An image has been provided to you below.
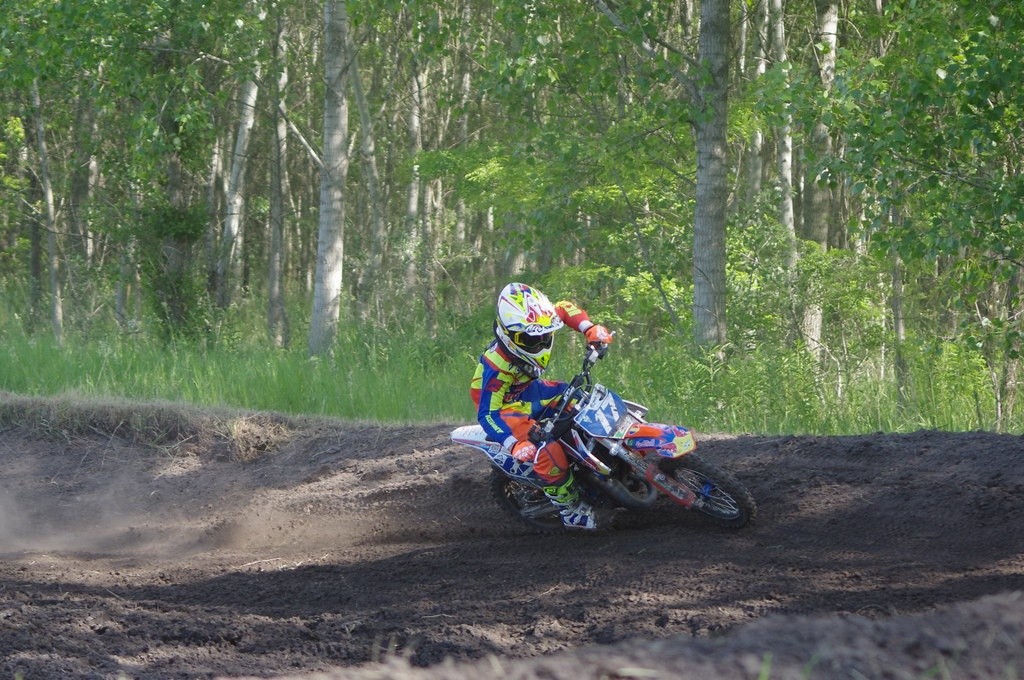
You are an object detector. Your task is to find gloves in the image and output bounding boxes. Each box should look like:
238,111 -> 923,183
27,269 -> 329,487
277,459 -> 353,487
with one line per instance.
511,440 -> 537,462
584,324 -> 613,344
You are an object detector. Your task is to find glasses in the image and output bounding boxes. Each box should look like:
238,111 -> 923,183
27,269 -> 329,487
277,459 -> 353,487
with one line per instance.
515,330 -> 555,348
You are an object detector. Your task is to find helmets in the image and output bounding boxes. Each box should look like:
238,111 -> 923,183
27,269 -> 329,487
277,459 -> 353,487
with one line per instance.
493,283 -> 564,370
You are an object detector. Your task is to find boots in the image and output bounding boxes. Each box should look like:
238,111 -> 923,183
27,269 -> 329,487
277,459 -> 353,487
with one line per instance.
543,470 -> 617,531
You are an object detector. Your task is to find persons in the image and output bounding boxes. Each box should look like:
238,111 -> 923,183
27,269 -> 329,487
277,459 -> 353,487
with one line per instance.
469,282 -> 612,532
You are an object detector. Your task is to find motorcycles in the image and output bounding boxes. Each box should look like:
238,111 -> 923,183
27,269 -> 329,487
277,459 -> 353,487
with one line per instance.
450,330 -> 758,537
